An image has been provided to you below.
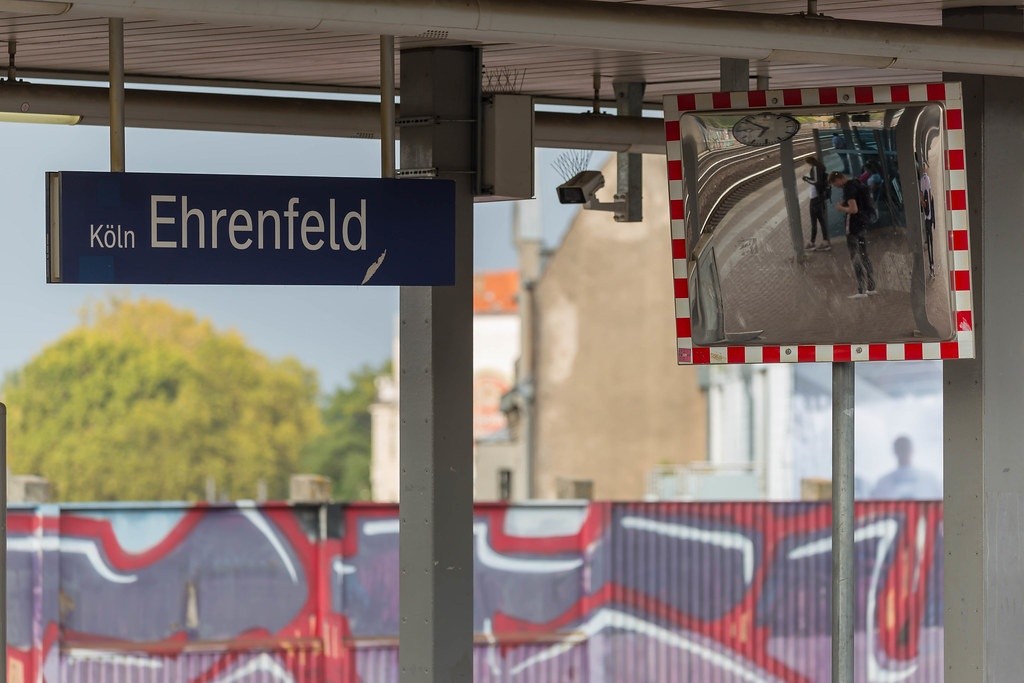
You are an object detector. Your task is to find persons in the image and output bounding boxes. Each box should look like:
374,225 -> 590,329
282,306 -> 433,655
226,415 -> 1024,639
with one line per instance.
921,164 -> 935,279
871,436 -> 943,500
833,131 -> 851,174
829,170 -> 879,297
802,156 -> 832,251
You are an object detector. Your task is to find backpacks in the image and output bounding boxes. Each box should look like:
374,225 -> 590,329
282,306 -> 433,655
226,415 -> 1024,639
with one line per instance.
850,181 -> 879,225
815,170 -> 831,201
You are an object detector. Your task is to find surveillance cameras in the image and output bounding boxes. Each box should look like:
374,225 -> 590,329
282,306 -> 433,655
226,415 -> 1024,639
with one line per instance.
557,171 -> 604,204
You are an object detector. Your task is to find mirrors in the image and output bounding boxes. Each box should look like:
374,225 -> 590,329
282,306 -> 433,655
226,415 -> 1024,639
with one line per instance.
663,81 -> 976,365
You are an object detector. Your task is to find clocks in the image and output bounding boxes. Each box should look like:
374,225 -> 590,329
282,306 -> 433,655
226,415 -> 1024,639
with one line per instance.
732,113 -> 799,147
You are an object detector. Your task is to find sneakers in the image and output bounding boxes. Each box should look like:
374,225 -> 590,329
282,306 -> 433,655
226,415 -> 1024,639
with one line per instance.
866,290 -> 877,296
803,243 -> 816,251
849,293 -> 867,300
815,243 -> 831,251
